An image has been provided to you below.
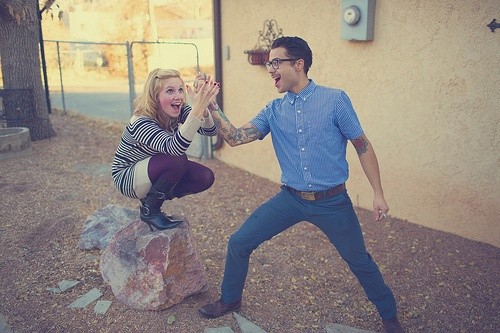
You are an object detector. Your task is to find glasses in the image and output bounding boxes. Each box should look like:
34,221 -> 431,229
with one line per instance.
264,58 -> 299,71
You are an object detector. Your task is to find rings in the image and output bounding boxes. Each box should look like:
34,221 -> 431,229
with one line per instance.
381,213 -> 387,219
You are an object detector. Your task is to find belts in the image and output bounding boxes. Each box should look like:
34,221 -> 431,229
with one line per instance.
287,182 -> 346,201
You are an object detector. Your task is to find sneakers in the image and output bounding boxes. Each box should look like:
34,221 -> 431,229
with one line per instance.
383,316 -> 404,333
197,298 -> 241,319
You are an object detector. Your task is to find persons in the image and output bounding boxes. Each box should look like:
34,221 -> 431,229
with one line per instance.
112,68 -> 221,232
193,37 -> 405,333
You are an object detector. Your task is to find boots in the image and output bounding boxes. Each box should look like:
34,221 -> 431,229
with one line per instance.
140,184 -> 182,232
